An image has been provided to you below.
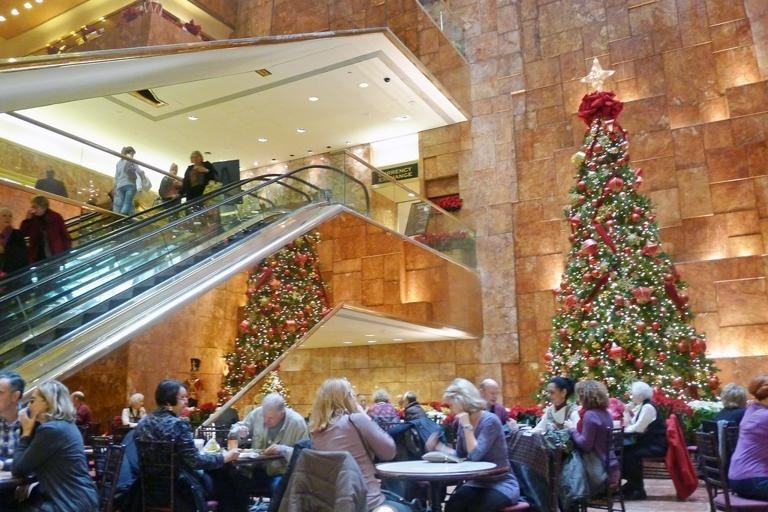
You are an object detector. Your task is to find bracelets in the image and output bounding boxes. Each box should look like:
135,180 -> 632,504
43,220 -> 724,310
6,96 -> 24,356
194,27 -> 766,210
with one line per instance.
462,424 -> 473,430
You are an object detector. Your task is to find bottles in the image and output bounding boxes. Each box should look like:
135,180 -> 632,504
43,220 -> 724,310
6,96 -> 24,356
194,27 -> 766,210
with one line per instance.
227,430 -> 239,451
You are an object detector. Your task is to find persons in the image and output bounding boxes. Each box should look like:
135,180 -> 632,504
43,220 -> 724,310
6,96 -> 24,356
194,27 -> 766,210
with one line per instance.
531,377 -> 666,512
479,378 -> 517,430
425,377 -> 520,512
308,378 -> 419,512
1,372 -> 100,512
0,207 -> 31,317
182,150 -> 218,230
36,167 -> 69,199
112,145 -> 147,215
706,375 -> 766,501
158,162 -> 183,223
20,195 -> 71,310
357,389 -> 428,423
110,379 -> 309,512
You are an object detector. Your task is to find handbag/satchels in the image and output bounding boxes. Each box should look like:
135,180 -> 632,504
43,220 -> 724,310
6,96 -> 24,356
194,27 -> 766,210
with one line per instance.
421,451 -> 466,463
201,180 -> 224,206
124,162 -> 137,181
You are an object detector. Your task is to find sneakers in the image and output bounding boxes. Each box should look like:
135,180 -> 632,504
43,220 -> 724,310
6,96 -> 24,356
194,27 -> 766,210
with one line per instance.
613,483 -> 645,499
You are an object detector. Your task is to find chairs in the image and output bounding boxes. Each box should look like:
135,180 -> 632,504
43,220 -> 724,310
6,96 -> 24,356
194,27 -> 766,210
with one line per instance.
135,438 -> 176,512
501,419 -> 768,512
89,436 -> 127,512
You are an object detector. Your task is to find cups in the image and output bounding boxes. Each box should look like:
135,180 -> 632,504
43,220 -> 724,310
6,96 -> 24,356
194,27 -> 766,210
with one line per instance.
613,420 -> 621,427
193,438 -> 204,451
